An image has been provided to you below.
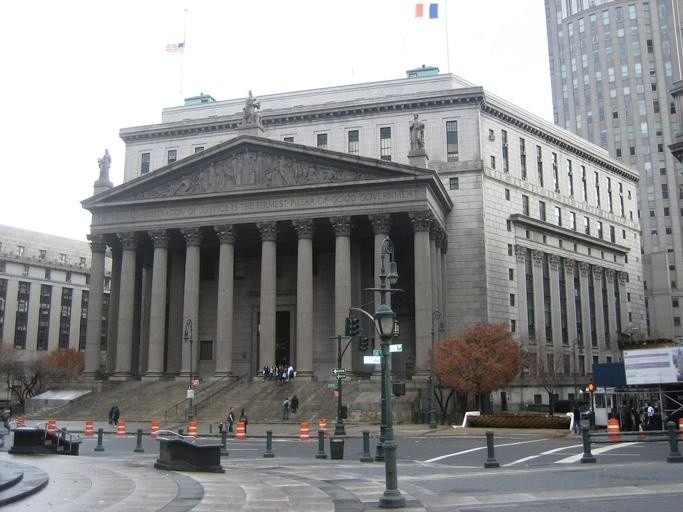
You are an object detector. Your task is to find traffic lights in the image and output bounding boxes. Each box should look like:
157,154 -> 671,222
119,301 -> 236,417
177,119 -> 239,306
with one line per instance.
587,383 -> 594,392
349,316 -> 359,336
362,337 -> 368,351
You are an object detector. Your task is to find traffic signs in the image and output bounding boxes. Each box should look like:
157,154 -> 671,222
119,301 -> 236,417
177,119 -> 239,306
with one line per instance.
330,368 -> 344,376
327,384 -> 339,389
335,373 -> 345,380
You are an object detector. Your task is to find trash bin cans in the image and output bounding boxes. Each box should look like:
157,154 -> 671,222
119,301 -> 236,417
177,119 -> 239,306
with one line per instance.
330,436 -> 344,459
417,412 -> 424,425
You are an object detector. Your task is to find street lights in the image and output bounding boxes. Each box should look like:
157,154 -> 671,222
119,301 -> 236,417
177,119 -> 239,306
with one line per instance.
572,338 -> 584,410
362,237 -> 405,463
183,318 -> 193,422
372,304 -> 406,510
429,309 -> 445,427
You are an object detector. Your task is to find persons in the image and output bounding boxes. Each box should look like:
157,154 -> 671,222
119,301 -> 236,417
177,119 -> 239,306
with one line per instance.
228,407 -> 235,432
621,400 -> 656,431
99,149 -> 112,179
282,397 -> 290,420
262,362 -> 296,382
410,113 -> 425,150
114,406 -> 120,425
239,408 -> 248,432
108,406 -> 115,424
245,90 -> 256,123
291,396 -> 299,413
571,404 -> 581,436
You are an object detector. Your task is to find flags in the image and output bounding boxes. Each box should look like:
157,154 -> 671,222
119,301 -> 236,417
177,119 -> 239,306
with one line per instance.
168,41 -> 185,55
416,3 -> 446,19
